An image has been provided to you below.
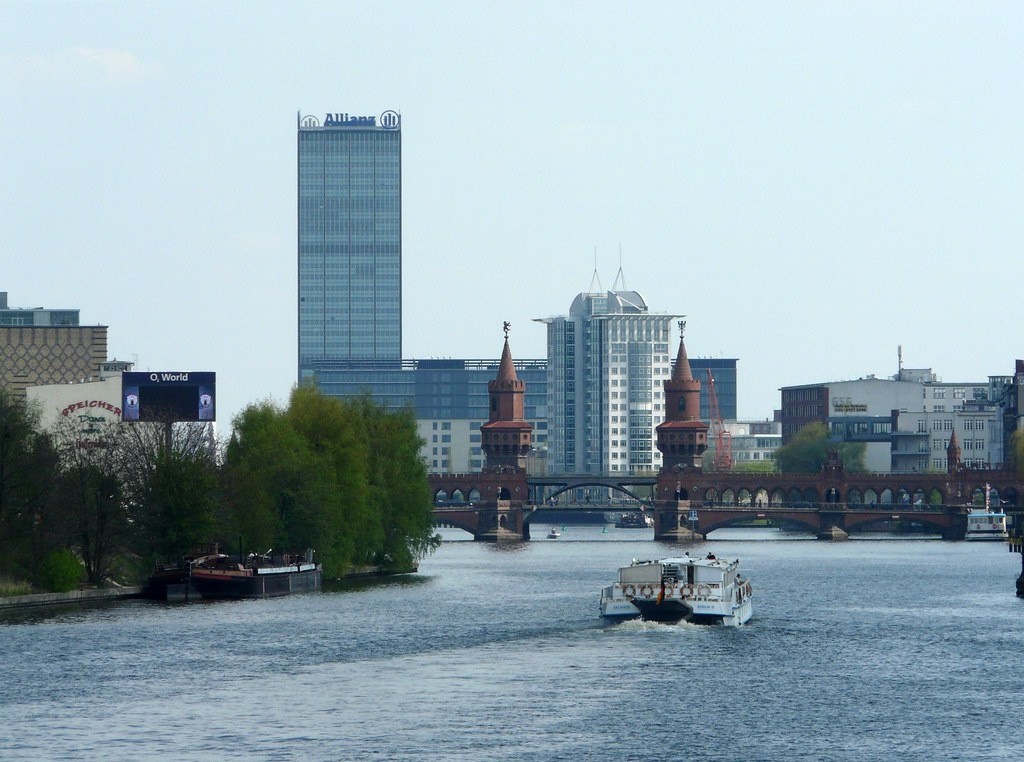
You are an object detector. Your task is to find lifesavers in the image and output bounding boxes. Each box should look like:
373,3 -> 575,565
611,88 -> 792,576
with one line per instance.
622,584 -> 637,597
680,584 -> 694,598
697,584 -> 712,598
664,585 -> 674,599
639,584 -> 654,599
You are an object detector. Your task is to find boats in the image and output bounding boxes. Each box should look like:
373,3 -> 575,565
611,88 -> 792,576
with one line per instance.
598,551 -> 754,629
615,511 -> 655,528
188,541 -> 326,600
546,525 -> 561,539
964,512 -> 1010,541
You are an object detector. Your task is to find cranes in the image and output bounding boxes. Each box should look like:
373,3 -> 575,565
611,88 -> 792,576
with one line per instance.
707,368 -> 733,472
